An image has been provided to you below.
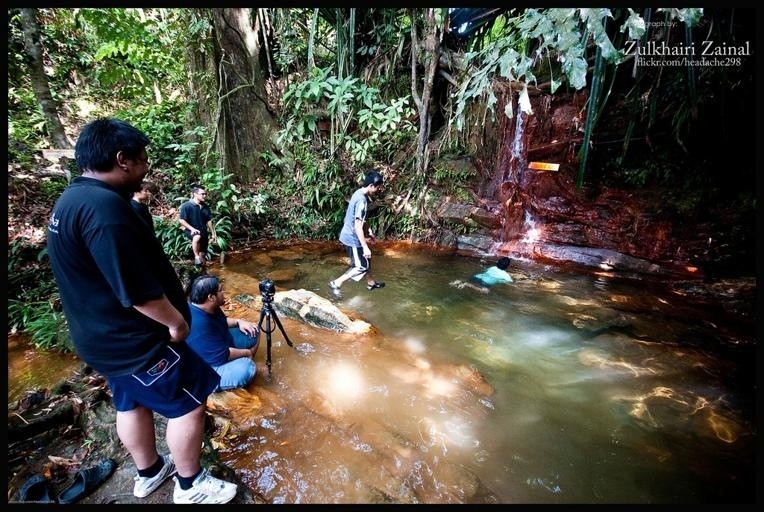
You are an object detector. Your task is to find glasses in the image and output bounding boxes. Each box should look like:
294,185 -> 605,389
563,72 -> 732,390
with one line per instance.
136,156 -> 151,168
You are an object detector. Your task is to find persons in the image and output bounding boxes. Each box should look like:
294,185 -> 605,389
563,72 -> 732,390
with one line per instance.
45,116 -> 241,508
448,255 -> 527,296
177,183 -> 217,271
183,276 -> 260,392
328,170 -> 387,301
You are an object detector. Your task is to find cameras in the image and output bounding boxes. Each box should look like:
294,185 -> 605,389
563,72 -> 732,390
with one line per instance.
256,278 -> 277,298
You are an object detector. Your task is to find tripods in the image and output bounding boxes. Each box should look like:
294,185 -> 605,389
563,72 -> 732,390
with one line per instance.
255,301 -> 296,380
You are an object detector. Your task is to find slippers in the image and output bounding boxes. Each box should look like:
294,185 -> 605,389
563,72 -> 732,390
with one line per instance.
18,474 -> 50,503
57,458 -> 116,504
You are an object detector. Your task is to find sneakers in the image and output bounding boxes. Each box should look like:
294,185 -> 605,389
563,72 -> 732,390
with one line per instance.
171,467 -> 239,504
327,280 -> 341,300
366,281 -> 385,290
193,256 -> 202,265
132,452 -> 178,498
